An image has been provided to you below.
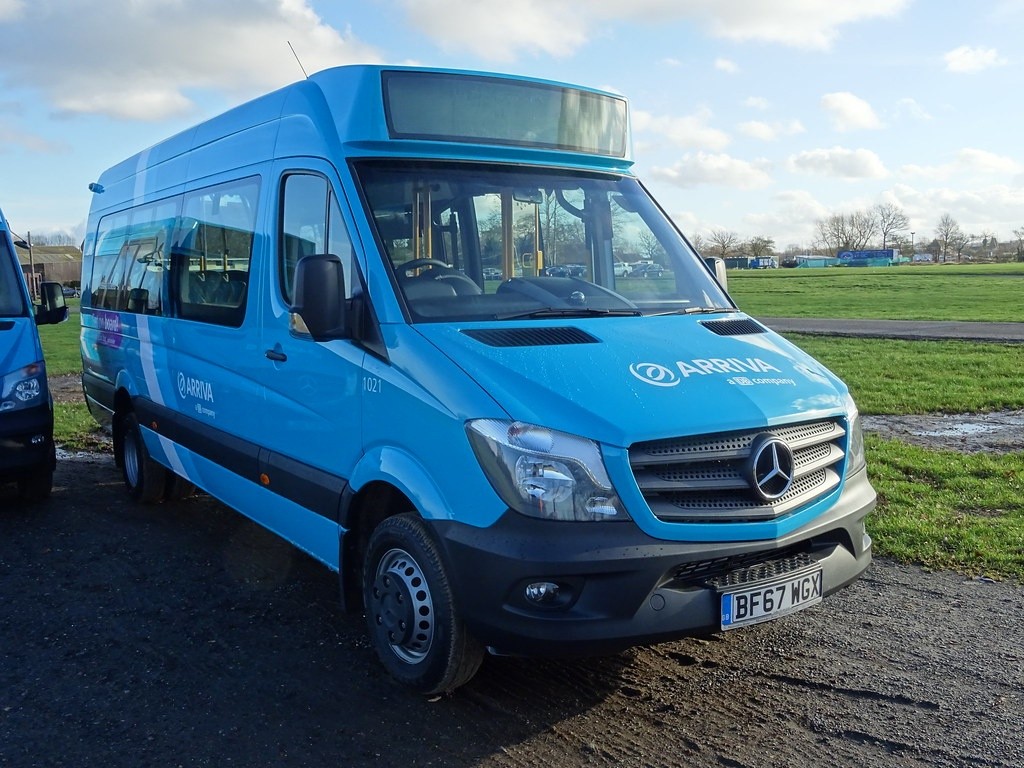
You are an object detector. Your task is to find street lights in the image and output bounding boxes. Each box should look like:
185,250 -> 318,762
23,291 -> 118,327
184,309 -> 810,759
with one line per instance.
911,232 -> 915,257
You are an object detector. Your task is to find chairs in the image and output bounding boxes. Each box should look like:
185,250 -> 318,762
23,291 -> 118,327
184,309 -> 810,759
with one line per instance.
192,271 -> 218,304
220,270 -> 248,305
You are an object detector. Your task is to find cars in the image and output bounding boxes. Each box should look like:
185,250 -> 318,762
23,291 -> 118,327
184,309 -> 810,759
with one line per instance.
939,256 -> 944,261
945,256 -> 951,260
482,268 -> 503,281
632,264 -> 664,278
1013,252 -> 1018,260
916,256 -> 930,262
405,271 -> 414,277
62,287 -> 80,298
459,269 -> 466,274
1001,252 -> 1014,261
545,264 -> 587,277
613,260 -> 632,278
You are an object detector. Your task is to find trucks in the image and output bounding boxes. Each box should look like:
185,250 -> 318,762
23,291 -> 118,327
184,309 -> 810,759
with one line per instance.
749,257 -> 772,268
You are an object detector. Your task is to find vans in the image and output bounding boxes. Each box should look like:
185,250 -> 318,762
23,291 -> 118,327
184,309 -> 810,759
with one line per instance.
78,63 -> 877,698
0,209 -> 69,509
91,216 -> 317,312
